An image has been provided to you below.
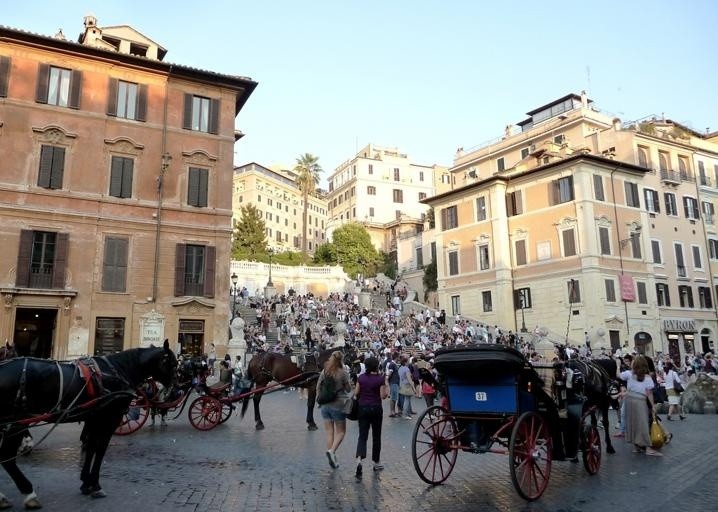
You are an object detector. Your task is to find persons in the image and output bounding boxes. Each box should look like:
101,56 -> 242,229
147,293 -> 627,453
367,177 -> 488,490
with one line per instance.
207,276 -> 717,478
146,379 -> 168,428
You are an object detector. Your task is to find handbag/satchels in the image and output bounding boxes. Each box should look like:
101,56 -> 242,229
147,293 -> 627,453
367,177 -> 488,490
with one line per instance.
651,385 -> 669,405
340,398 -> 358,421
650,421 -> 664,449
673,380 -> 684,393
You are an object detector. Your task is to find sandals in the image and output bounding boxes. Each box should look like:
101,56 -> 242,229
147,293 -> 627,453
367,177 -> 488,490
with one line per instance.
645,450 -> 664,457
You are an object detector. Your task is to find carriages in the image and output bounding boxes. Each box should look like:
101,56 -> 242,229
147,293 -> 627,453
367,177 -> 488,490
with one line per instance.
114,339 -> 361,435
413,344 -> 632,501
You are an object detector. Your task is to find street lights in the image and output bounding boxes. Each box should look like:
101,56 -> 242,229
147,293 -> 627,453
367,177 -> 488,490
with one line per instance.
232,272 -> 238,317
361,257 -> 366,286
267,247 -> 274,286
520,292 -> 527,331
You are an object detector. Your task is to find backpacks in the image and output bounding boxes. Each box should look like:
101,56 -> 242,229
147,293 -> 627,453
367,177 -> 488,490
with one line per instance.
316,369 -> 344,404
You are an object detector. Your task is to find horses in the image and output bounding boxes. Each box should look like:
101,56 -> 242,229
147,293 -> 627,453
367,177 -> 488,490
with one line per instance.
0,338 -> 188,512
560,356 -> 633,465
238,342 -> 361,431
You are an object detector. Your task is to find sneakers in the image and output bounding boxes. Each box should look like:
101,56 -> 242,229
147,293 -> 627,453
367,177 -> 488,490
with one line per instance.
222,405 -> 239,417
388,411 -> 437,420
147,422 -> 168,427
372,464 -> 384,471
325,449 -> 340,469
353,463 -> 362,479
597,420 -> 625,438
